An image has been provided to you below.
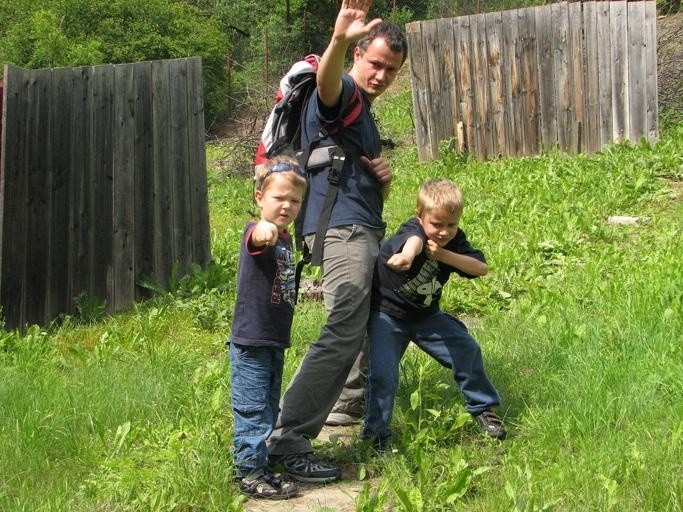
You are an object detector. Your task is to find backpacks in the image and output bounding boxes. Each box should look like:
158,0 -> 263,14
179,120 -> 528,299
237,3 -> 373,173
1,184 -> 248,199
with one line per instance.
255,54 -> 362,169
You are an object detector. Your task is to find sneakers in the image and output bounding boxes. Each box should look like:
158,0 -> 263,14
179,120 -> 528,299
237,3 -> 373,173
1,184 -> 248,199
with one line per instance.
239,454 -> 341,500
326,406 -> 361,425
474,411 -> 507,438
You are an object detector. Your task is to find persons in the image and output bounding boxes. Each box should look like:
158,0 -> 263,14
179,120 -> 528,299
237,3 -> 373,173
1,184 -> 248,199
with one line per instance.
267,0 -> 408,484
229,155 -> 310,500
360,177 -> 506,459
